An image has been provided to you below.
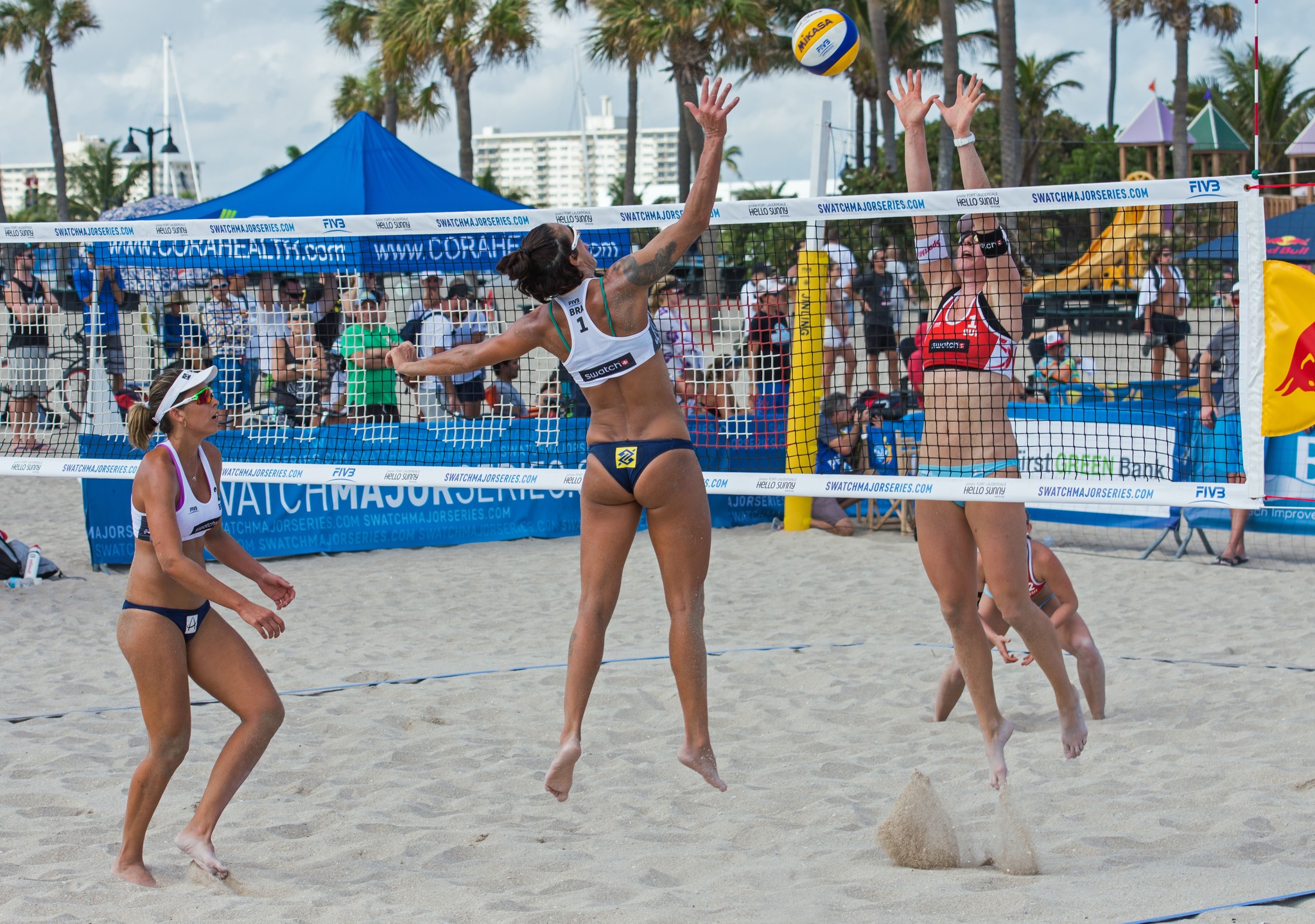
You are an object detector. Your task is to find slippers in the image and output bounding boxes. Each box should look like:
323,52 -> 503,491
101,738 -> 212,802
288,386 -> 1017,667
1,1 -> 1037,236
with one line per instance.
771,517 -> 784,534
1231,555 -> 1249,567
1210,556 -> 1233,566
10,447 -> 32,453
30,444 -> 55,451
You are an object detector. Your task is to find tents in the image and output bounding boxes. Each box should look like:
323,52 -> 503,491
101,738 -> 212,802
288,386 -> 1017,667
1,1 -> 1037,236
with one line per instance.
73,109 -> 635,432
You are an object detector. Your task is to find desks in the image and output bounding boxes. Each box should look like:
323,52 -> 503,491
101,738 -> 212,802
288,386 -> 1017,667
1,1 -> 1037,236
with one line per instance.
1024,290 -> 1139,336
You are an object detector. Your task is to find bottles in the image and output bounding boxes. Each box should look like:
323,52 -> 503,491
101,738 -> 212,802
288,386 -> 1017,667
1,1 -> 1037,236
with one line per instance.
23,545 -> 41,578
4,577 -> 42,589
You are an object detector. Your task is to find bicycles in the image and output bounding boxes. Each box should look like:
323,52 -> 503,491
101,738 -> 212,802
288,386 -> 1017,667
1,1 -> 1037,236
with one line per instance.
0,319 -> 89,423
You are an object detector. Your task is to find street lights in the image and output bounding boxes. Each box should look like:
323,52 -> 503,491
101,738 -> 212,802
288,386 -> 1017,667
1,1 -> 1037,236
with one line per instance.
123,124 -> 180,197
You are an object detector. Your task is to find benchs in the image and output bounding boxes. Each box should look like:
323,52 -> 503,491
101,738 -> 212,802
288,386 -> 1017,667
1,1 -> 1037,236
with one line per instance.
909,309 -> 1135,320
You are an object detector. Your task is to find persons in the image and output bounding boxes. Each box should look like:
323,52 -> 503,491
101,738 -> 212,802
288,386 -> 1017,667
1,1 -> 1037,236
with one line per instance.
116,363 -> 299,890
1038,329 -> 1083,389
72,245 -> 126,407
1008,373 -> 1047,403
1135,244 -> 1191,381
2,242 -> 58,453
381,77 -> 742,807
771,392 -> 884,536
647,227 -> 917,419
932,509 -> 1106,723
1200,264 -> 1249,567
157,272 -> 562,426
885,68 -> 1088,791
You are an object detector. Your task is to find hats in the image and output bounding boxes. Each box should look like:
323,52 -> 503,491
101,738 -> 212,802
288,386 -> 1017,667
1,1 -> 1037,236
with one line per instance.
867,247 -> 881,270
152,365 -> 218,422
446,284 -> 482,304
751,262 -> 776,276
354,291 -> 377,306
421,270 -> 444,279
956,213 -> 1012,254
659,279 -> 691,292
753,278 -> 786,298
1229,281 -> 1239,294
1043,332 -> 1064,350
164,292 -> 194,308
717,357 -> 748,369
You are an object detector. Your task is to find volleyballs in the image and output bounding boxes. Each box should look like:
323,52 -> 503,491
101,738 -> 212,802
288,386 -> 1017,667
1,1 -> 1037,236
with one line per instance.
791,8 -> 860,76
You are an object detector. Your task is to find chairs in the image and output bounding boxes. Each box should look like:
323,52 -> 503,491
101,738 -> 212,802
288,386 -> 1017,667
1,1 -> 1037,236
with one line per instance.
147,299 -> 197,374
853,425 -> 921,536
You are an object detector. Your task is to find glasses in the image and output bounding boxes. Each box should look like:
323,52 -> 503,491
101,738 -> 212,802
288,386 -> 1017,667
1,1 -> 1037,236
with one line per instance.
463,298 -> 473,308
425,278 -> 440,283
210,283 -> 228,289
876,256 -> 888,260
282,291 -> 304,299
18,253 -> 36,259
1231,299 -> 1240,306
764,293 -> 779,298
1056,343 -> 1066,347
161,387 -> 212,415
289,315 -> 311,321
958,231 -> 980,244
565,224 -> 580,252
662,288 -> 684,293
1161,253 -> 1171,257
831,267 -> 843,271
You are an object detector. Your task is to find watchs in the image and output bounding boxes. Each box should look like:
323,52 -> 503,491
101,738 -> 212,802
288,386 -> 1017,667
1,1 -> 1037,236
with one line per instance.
952,130 -> 976,147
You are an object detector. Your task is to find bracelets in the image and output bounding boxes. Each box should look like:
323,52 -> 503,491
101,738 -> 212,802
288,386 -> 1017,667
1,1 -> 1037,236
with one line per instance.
292,362 -> 296,371
913,230 -> 949,264
974,224 -> 1010,260
37,303 -> 42,313
110,279 -> 116,284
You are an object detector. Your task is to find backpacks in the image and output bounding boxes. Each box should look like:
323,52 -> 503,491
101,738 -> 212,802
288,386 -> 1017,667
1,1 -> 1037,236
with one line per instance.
399,308 -> 449,358
113,384 -> 150,426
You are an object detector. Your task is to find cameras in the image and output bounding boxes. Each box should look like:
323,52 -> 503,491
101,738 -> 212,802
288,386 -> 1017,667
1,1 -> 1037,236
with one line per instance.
852,403 -> 907,422
